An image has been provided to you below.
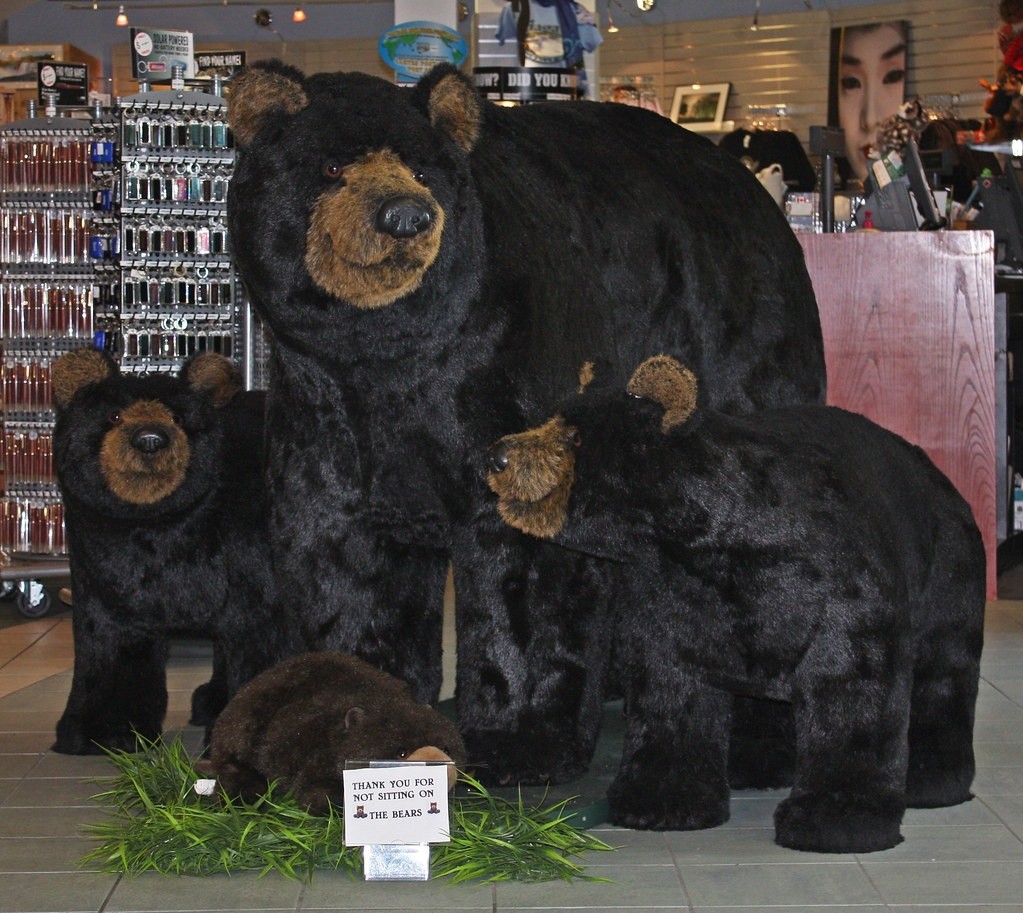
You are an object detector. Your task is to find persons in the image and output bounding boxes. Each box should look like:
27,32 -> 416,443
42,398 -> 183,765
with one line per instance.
838,17 -> 907,179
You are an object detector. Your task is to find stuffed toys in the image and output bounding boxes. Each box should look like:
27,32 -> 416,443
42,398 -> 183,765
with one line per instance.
497,354 -> 985,851
219,60 -> 824,791
195,649 -> 469,825
46,347 -> 290,761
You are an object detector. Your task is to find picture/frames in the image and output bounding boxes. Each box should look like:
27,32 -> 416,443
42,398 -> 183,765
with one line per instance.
665,80 -> 731,135
827,20 -> 913,186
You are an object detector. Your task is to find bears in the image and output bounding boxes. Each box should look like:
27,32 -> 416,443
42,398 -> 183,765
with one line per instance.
47,59 -> 989,856
